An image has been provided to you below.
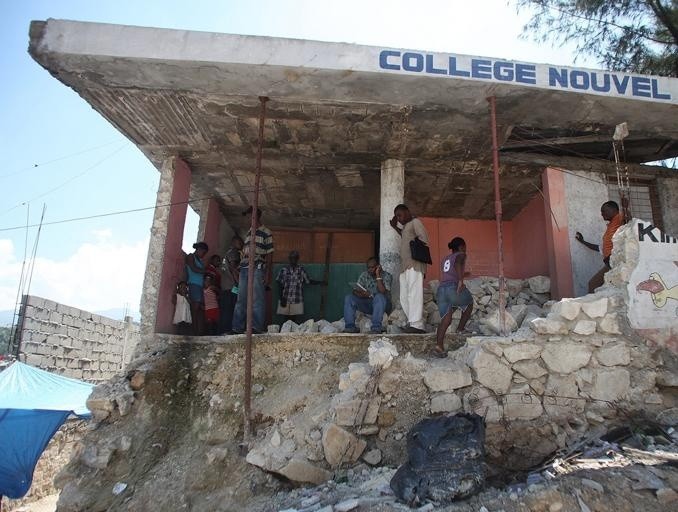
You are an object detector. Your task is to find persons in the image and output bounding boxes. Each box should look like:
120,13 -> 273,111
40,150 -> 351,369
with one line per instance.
574,199 -> 633,295
338,255 -> 394,334
431,236 -> 474,358
183,241 -> 216,336
275,249 -> 325,326
219,235 -> 245,335
225,204 -> 275,335
388,203 -> 433,333
198,273 -> 225,336
170,281 -> 195,336
204,254 -> 221,290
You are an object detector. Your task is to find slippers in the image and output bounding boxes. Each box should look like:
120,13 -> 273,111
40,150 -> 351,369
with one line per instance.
432,345 -> 448,358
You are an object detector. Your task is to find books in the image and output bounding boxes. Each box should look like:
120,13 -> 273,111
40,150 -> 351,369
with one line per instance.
347,282 -> 374,299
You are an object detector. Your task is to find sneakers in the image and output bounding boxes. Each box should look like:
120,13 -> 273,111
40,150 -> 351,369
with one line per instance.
342,326 -> 475,335
226,328 -> 263,335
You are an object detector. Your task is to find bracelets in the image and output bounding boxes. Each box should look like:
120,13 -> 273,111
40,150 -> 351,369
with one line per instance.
376,278 -> 383,280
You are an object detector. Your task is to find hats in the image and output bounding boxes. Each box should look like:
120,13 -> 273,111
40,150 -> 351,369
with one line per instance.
241,205 -> 262,217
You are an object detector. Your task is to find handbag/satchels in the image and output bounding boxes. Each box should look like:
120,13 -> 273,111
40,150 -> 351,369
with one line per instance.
409,236 -> 433,265
